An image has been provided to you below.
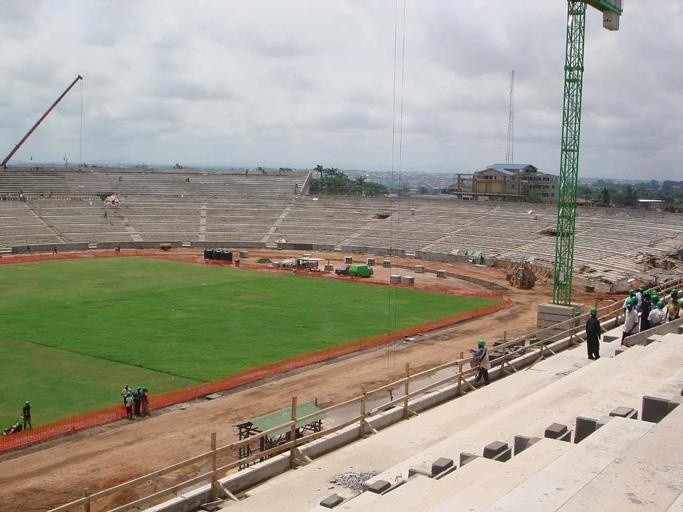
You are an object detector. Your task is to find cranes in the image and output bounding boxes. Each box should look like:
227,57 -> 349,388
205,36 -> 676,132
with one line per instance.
552,0 -> 623,307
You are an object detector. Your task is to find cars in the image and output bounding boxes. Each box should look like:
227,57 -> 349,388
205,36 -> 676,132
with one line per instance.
335,264 -> 351,275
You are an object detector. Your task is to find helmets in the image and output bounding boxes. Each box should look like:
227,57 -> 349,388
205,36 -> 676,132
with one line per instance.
639,285 -> 659,302
657,301 -> 665,308
670,289 -> 678,295
479,340 -> 486,346
591,308 -> 597,314
629,289 -> 636,295
625,297 -> 637,307
677,298 -> 683,306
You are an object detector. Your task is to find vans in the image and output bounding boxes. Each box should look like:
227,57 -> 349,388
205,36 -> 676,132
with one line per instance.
349,264 -> 374,277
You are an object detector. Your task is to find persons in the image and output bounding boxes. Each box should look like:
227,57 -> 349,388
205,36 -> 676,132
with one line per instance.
586,308 -> 601,359
121,384 -> 151,420
23,401 -> 32,430
4,421 -> 22,435
621,285 -> 680,344
469,340 -> 489,385
